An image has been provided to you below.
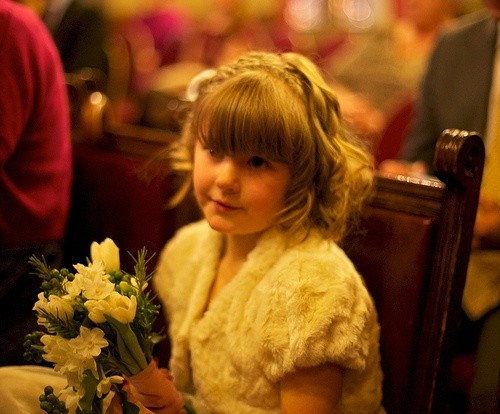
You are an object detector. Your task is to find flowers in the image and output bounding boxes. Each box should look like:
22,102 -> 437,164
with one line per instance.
24,237 -> 182,414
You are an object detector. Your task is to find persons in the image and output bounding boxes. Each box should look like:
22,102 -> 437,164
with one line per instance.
0,0 -> 73,296
378,0 -> 500,414
0,51 -> 388,414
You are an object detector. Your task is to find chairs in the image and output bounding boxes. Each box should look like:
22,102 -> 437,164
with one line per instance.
63,91 -> 485,414
